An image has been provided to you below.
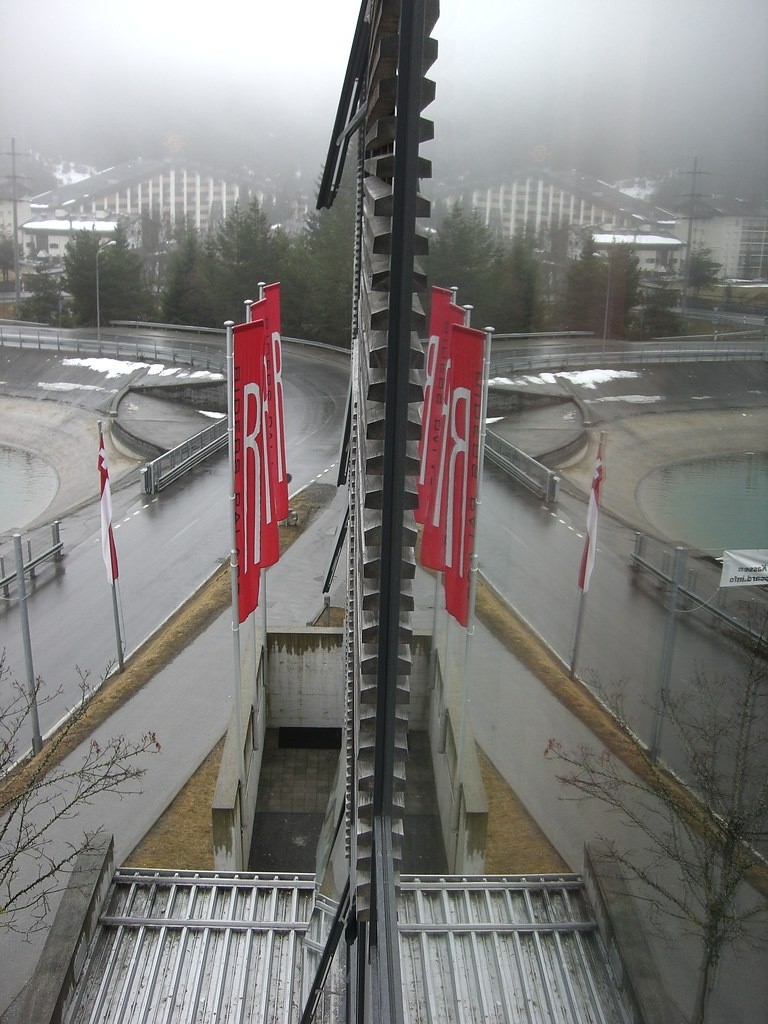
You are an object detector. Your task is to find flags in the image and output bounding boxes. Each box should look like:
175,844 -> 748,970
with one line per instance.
578,444 -> 604,596
225,281 -> 290,624
97,433 -> 120,584
413,282 -> 486,626
